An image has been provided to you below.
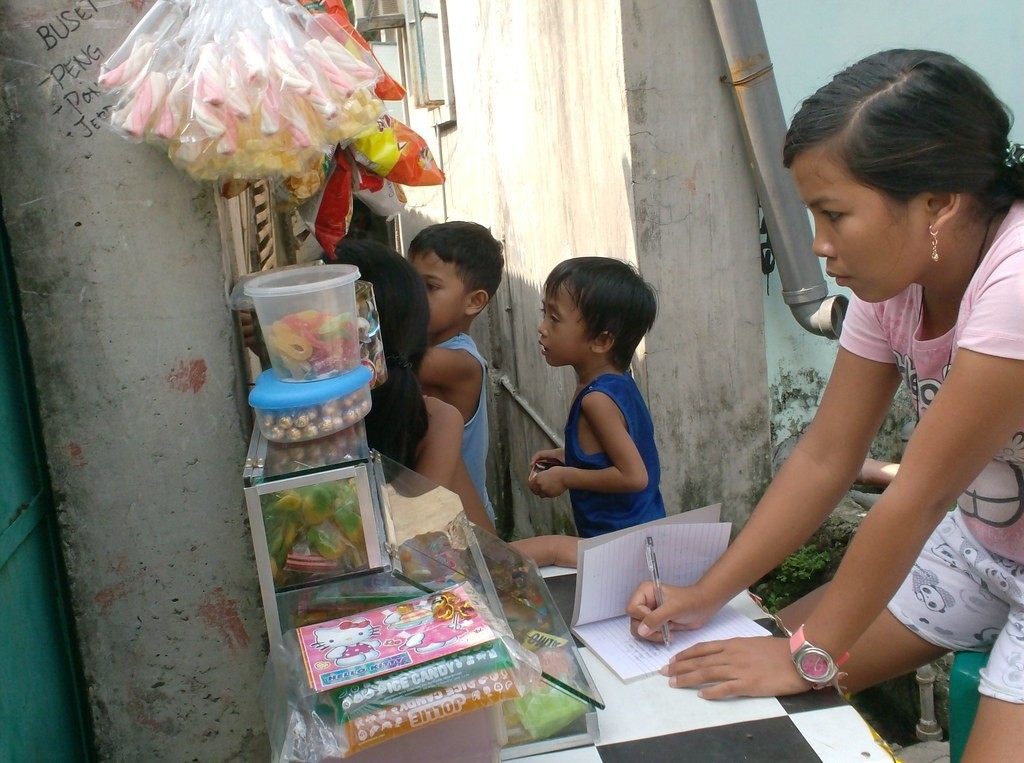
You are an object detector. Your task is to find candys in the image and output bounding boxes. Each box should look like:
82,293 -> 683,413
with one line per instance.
263,309 -> 369,586
101,9 -> 381,182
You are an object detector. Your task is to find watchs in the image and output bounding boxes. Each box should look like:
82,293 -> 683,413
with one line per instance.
790,622 -> 838,686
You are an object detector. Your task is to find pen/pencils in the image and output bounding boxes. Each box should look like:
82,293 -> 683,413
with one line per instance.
646,536 -> 669,647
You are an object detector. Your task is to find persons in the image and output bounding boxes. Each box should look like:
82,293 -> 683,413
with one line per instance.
625,47 -> 1024,763
527,257 -> 667,536
406,221 -> 504,526
853,459 -> 990,763
240,238 -> 588,569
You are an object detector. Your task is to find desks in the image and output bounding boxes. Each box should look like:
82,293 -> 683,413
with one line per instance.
493,564 -> 904,763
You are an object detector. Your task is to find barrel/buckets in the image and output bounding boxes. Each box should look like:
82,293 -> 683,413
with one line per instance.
354,279 -> 388,390
243,264 -> 361,380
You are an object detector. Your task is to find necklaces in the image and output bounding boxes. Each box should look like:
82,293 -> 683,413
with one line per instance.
914,209 -> 994,427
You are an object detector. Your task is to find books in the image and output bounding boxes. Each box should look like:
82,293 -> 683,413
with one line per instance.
571,503 -> 774,684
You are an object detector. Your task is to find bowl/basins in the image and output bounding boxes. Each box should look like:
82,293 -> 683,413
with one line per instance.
247,363 -> 377,442
